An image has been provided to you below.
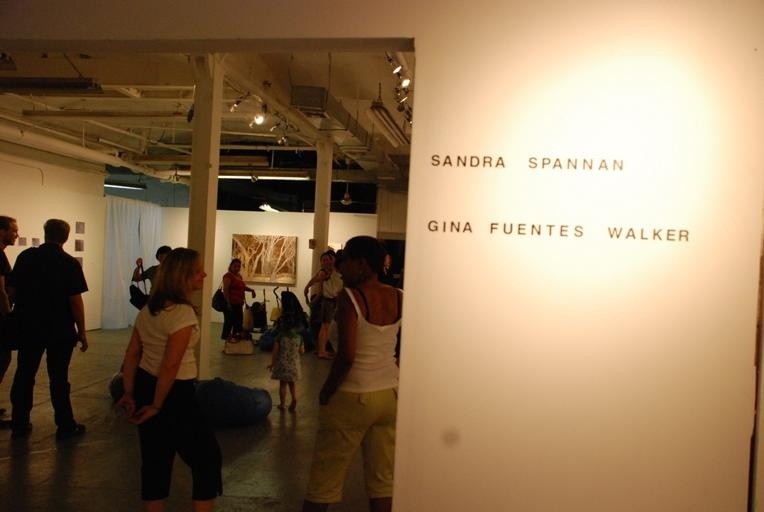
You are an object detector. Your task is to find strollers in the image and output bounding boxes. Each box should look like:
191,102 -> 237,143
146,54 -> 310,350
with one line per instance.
245,285 -> 307,332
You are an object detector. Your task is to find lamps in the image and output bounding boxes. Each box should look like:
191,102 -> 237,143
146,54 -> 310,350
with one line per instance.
228,93 -> 289,145
385,57 -> 412,120
258,192 -> 289,212
366,82 -> 410,148
104,173 -> 148,190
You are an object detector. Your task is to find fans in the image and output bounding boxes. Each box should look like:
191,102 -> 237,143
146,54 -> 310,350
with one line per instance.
331,158 -> 378,205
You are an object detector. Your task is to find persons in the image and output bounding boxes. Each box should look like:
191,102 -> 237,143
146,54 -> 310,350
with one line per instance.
264,310 -> 305,411
297,235 -> 404,510
114,246 -> 224,511
130,245 -> 172,292
219,257 -> 256,343
302,246 -> 402,360
0,217 -> 91,442
260,288 -> 310,341
0,214 -> 21,429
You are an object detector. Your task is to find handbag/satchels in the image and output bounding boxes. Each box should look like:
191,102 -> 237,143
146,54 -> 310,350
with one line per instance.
212,287 -> 227,313
224,339 -> 256,356
130,284 -> 150,310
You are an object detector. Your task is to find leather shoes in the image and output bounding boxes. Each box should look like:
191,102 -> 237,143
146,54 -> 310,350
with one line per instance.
12,423 -> 33,440
57,419 -> 86,440
